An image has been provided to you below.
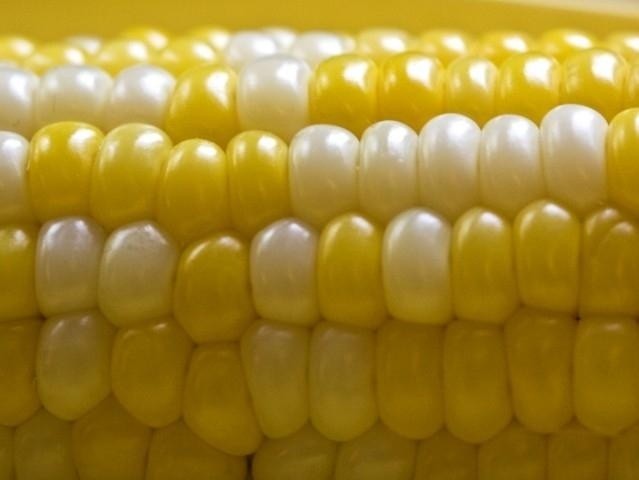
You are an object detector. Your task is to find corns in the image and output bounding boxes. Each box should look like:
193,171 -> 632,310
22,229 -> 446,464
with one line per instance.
1,28 -> 639,479
